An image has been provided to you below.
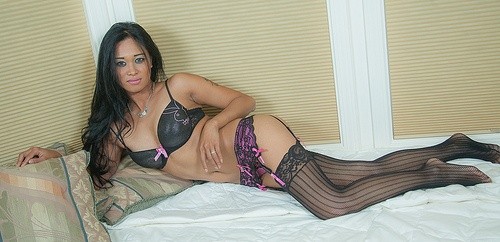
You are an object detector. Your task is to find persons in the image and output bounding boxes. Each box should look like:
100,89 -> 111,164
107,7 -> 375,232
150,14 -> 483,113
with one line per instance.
8,21 -> 500,223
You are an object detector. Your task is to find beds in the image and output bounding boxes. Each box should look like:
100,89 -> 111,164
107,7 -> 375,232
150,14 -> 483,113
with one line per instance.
0,133 -> 500,242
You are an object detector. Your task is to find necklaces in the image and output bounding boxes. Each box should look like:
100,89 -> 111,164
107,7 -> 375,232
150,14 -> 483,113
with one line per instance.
126,86 -> 153,118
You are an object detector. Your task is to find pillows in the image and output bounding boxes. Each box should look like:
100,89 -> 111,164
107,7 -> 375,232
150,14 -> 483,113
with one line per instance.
91,154 -> 210,229
0,150 -> 111,242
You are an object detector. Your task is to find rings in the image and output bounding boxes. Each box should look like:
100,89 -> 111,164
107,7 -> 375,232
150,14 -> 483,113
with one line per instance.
210,150 -> 216,155
